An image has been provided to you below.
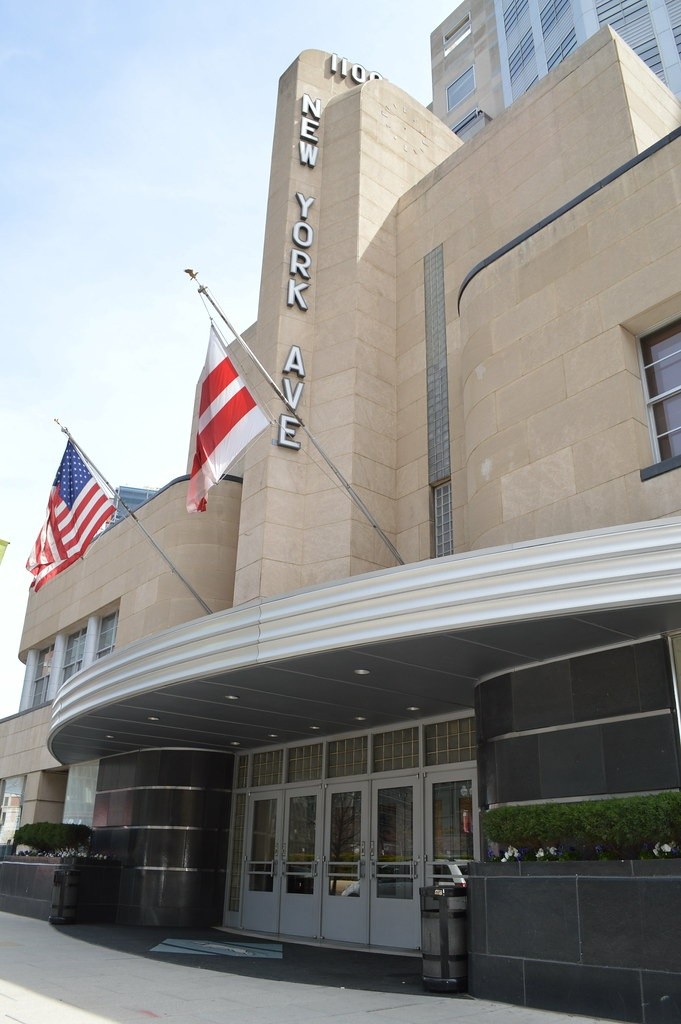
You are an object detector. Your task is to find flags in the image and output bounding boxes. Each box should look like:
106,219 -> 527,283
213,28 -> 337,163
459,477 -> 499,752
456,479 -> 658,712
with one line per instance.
25,439 -> 118,594
186,319 -> 274,514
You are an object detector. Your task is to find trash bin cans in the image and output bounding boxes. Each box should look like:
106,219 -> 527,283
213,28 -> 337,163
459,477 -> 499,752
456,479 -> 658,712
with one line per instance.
48,870 -> 79,926
417,885 -> 470,995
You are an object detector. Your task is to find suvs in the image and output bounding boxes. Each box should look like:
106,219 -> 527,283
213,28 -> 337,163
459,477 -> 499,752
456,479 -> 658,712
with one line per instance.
342,859 -> 472,897
